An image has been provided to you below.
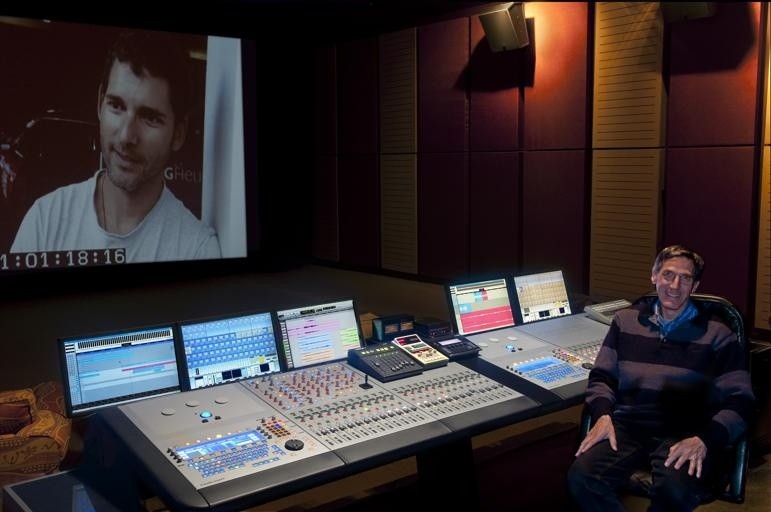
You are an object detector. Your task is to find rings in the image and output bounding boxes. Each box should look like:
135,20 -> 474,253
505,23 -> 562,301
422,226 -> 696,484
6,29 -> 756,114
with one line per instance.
690,457 -> 698,463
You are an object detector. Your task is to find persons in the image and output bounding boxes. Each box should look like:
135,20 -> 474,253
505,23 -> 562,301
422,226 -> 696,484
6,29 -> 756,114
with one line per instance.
5,28 -> 224,260
568,243 -> 759,512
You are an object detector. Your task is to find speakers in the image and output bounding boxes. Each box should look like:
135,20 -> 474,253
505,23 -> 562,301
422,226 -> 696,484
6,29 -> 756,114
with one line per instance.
478,1 -> 530,53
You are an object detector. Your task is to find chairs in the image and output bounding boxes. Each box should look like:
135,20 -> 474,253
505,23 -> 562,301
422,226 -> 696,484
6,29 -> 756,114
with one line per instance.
581,293 -> 753,504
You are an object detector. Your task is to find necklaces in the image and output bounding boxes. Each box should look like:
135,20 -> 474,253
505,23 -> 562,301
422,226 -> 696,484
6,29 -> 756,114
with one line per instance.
95,169 -> 108,232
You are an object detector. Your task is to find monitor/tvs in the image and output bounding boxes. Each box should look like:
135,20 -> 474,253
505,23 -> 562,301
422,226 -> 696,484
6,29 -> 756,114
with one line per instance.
445,275 -> 520,336
274,297 -> 366,372
178,309 -> 285,392
60,324 -> 184,418
510,267 -> 575,326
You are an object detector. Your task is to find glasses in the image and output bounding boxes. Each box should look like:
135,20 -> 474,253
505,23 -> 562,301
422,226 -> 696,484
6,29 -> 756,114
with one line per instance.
658,269 -> 694,287
